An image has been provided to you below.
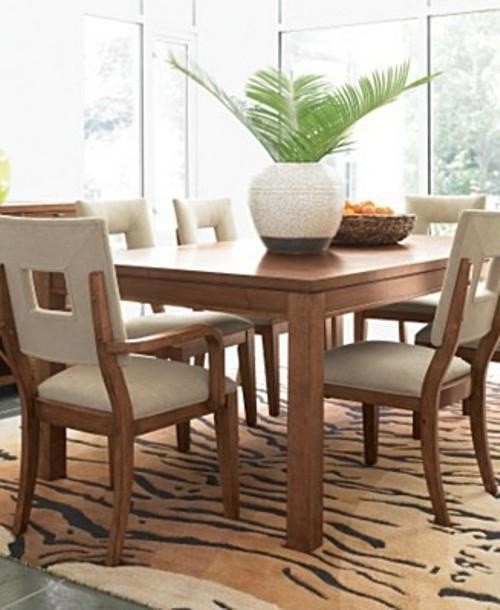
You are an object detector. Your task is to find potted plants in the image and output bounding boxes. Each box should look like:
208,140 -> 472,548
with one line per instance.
165,50 -> 443,255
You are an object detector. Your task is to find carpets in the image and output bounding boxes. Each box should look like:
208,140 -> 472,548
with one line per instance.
0,369 -> 500,610
